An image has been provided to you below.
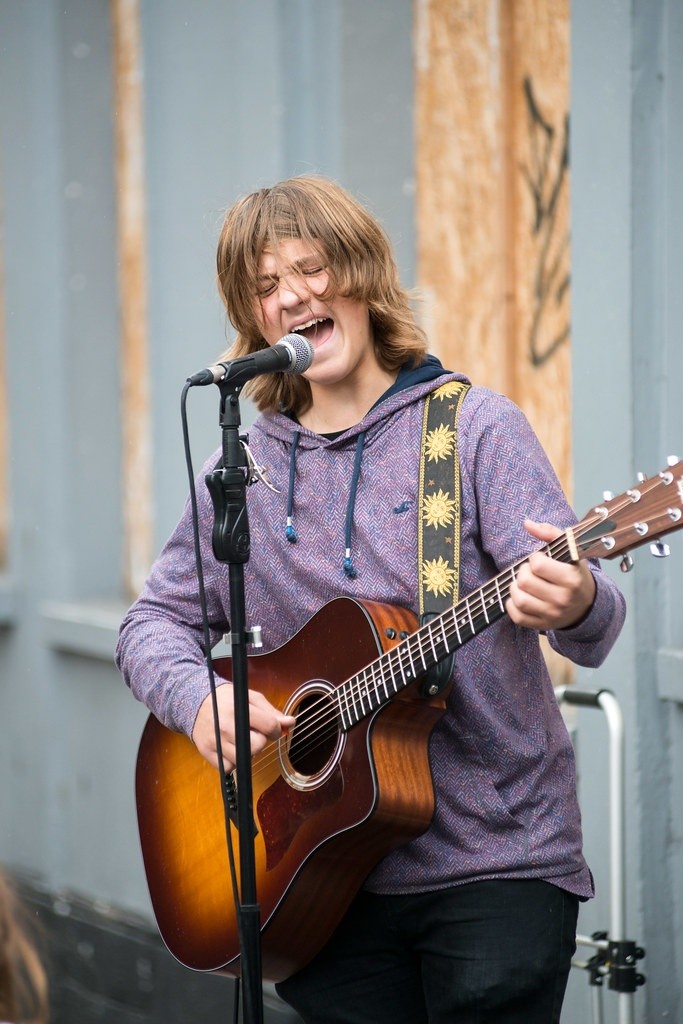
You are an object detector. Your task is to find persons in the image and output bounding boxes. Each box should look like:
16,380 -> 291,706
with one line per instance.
116,176 -> 628,1024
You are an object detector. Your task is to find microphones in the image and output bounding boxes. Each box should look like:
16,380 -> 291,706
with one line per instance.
186,334 -> 315,385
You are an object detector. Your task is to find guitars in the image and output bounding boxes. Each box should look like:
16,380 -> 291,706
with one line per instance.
131,451 -> 683,978
